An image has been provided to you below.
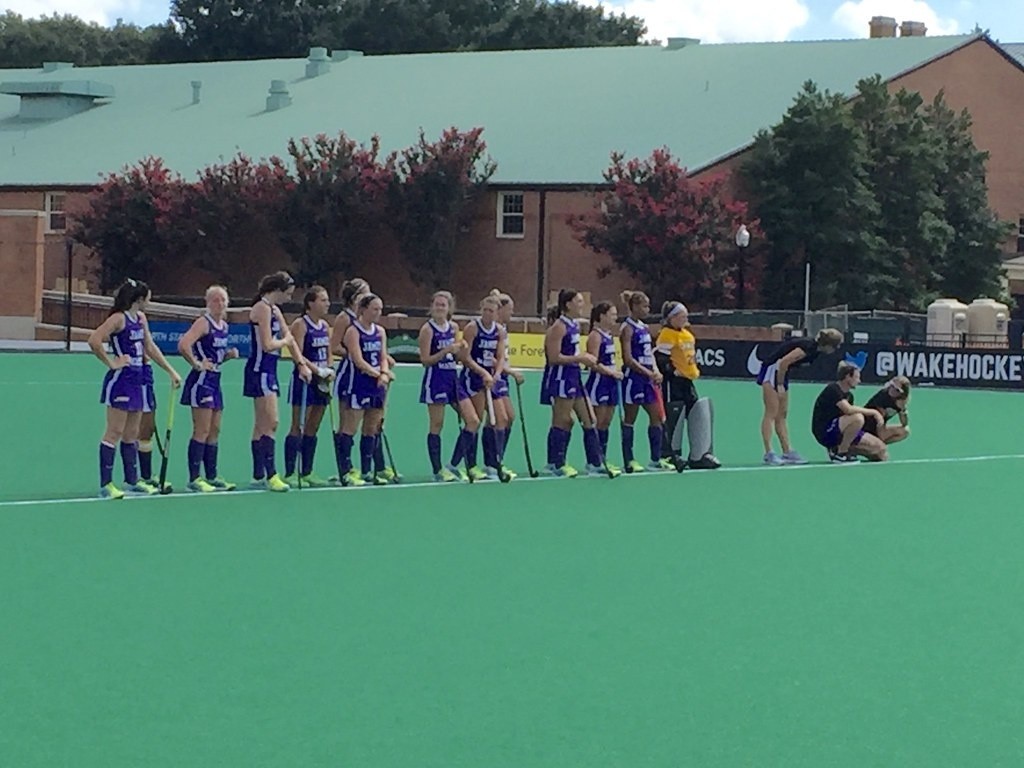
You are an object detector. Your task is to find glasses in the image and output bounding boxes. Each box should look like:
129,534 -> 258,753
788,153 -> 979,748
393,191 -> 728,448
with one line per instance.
890,382 -> 903,393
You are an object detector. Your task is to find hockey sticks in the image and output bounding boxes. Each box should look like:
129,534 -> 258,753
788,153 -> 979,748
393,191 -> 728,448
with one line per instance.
452,354 -> 477,483
484,384 -> 513,483
326,378 -> 352,487
298,381 -> 311,489
516,383 -> 539,477
651,380 -> 688,473
580,380 -> 622,479
373,380 -> 400,485
157,388 -> 176,494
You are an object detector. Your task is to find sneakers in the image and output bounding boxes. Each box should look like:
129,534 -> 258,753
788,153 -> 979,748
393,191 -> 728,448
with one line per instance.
623,446 -> 860,474
300,470 -> 327,486
446,461 -> 469,482
361,471 -> 388,484
553,463 -> 578,477
186,475 -> 216,492
377,467 -> 403,480
98,482 -> 124,499
264,473 -> 290,491
585,461 -> 621,476
123,480 -> 158,495
145,474 -> 172,494
466,465 -> 487,479
282,473 -> 310,488
487,464 -> 517,477
543,464 -> 553,473
205,477 -> 236,490
432,468 -> 457,482
482,466 -> 498,478
248,478 -> 268,489
340,468 -> 366,485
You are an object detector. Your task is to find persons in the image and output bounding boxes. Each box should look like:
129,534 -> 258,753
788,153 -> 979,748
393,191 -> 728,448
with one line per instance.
862,375 -> 911,444
540,288 -> 720,476
330,278 -> 402,486
177,286 -> 238,492
242,271 -> 312,492
445,288 -> 523,481
812,361 -> 888,465
756,329 -> 842,465
418,290 -> 480,482
88,279 -> 181,500
282,285 -> 330,489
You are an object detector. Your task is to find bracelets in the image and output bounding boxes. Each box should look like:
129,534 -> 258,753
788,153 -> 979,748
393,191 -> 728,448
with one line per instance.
777,382 -> 784,385
297,361 -> 305,365
